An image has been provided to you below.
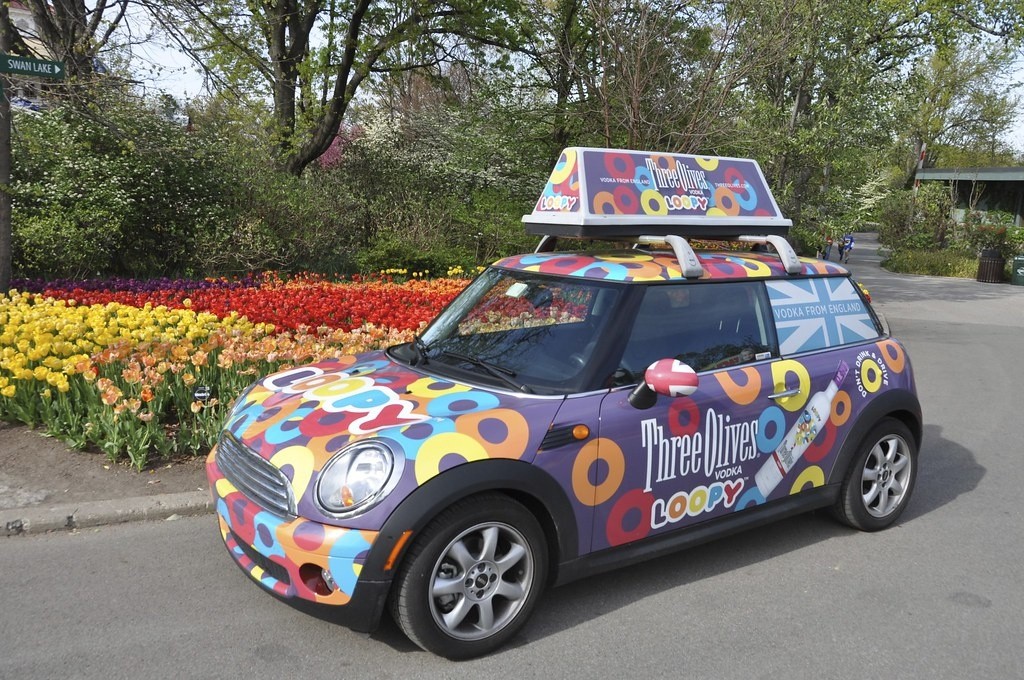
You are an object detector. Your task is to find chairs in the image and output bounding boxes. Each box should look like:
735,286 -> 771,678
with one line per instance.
680,288 -> 759,376
623,291 -> 679,374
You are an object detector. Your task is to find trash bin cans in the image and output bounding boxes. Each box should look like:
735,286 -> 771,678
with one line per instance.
977,249 -> 1006,282
1012,254 -> 1024,286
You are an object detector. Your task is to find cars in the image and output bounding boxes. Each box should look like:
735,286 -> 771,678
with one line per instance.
205,145 -> 923,662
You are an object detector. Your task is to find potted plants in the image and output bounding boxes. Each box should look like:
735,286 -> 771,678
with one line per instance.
963,209 -> 1024,283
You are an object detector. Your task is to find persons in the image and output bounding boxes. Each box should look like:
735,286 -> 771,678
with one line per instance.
821,228 -> 833,260
838,235 -> 844,260
843,231 -> 855,264
